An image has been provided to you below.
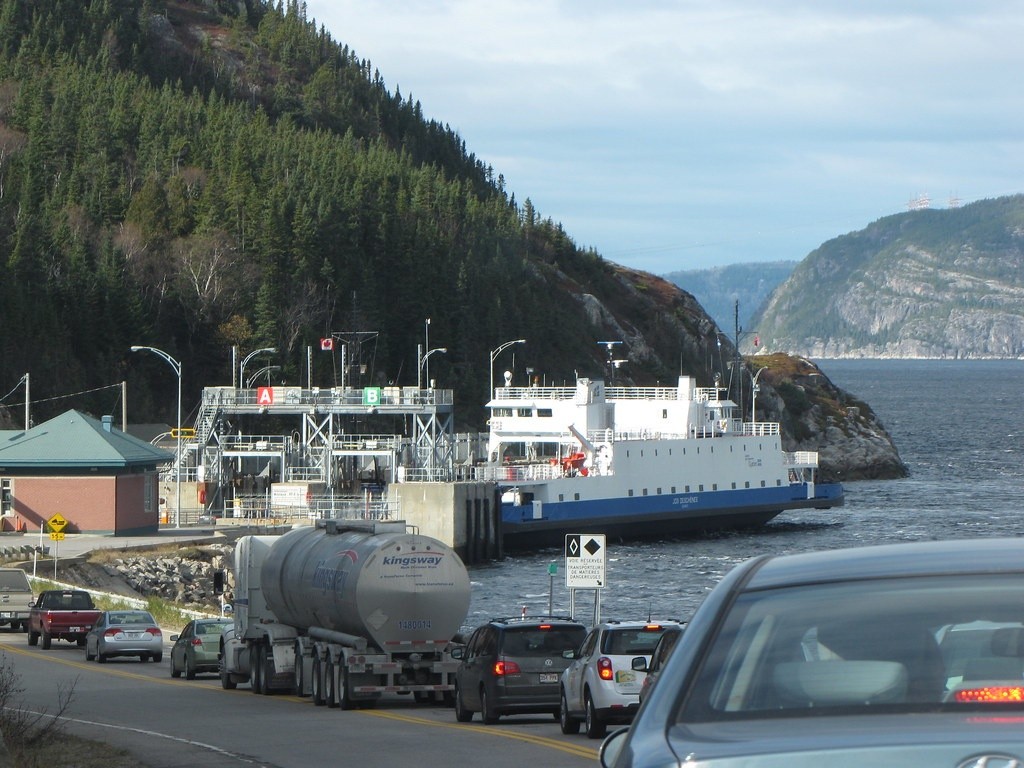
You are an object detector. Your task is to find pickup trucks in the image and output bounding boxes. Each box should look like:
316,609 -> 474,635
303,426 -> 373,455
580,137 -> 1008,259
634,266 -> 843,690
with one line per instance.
27,590 -> 103,650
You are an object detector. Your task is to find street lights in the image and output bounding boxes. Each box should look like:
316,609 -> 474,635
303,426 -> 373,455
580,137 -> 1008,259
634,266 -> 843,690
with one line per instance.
240,347 -> 277,390
131,345 -> 183,529
597,340 -> 623,384
751,365 -> 774,439
247,365 -> 282,403
490,339 -> 529,406
416,344 -> 448,402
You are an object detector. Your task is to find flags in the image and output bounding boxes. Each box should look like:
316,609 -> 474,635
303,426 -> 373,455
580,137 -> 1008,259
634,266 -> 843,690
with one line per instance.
717,337 -> 721,350
320,337 -> 334,350
754,336 -> 758,347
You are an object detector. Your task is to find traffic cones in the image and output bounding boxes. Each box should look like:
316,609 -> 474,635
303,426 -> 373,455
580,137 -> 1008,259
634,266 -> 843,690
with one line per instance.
16,518 -> 23,532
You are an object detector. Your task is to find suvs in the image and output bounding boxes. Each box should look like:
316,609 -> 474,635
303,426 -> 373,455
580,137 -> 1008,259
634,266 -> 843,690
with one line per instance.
558,619 -> 693,738
451,614 -> 591,724
0,567 -> 38,634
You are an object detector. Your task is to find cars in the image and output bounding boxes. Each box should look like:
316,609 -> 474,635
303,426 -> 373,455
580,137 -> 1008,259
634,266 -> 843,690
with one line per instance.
929,622 -> 1024,703
169,618 -> 236,680
84,610 -> 165,663
600,540 -> 1024,768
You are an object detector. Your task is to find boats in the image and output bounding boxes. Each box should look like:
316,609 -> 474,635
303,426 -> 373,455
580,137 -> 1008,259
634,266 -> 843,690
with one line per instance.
549,452 -> 589,470
471,298 -> 847,543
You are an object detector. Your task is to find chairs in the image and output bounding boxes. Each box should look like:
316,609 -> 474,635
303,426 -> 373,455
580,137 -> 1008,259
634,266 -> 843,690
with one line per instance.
544,633 -> 570,656
135,618 -> 145,623
505,631 -> 524,655
771,660 -> 907,705
611,636 -> 633,655
71,598 -> 81,609
47,598 -> 59,609
126,619 -> 133,623
961,627 -> 1024,683
110,618 -> 120,624
196,625 -> 206,634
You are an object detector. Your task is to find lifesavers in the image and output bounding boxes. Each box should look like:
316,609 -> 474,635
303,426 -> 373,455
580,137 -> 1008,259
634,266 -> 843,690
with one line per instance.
200,490 -> 206,504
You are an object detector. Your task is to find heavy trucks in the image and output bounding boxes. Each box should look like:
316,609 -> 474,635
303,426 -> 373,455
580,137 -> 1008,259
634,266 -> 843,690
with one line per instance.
213,516 -> 471,714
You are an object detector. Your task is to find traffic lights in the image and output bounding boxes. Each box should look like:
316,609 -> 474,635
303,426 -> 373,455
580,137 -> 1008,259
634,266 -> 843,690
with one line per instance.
170,429 -> 195,438
